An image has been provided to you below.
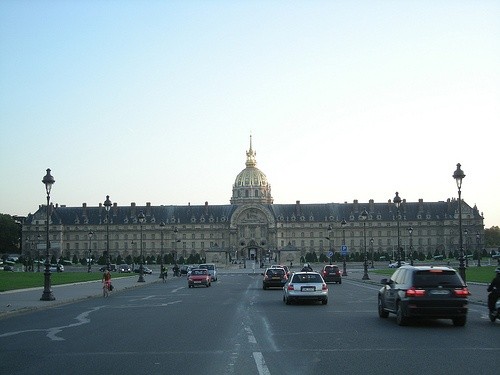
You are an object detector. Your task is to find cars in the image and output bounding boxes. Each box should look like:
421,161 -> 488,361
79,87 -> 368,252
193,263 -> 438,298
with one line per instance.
0,256 -> 64,273
134,266 -> 152,274
261,265 -> 290,289
181,265 -> 198,275
188,269 -> 211,288
118,264 -> 131,273
389,261 -> 409,268
283,271 -> 328,304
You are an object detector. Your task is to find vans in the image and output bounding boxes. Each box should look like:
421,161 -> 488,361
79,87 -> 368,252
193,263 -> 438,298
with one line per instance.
199,264 -> 217,282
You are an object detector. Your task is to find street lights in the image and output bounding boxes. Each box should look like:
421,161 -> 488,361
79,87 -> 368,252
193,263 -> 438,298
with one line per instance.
137,213 -> 179,282
41,168 -> 56,301
327,207 -> 371,280
87,195 -> 113,272
393,192 -> 414,268
452,163 -> 481,285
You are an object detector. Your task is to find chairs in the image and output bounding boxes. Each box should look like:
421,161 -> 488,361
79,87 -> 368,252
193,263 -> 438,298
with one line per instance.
294,276 -> 300,283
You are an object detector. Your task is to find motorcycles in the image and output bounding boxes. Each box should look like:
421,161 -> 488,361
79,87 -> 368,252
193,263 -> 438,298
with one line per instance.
489,281 -> 500,321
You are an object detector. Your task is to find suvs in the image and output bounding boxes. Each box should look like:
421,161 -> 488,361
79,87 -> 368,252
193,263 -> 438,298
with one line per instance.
378,265 -> 469,327
322,265 -> 342,284
99,264 -> 117,272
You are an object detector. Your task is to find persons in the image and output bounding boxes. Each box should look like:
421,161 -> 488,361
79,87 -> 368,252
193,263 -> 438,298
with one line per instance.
158,264 -> 180,277
290,262 -> 314,272
486,268 -> 500,315
101,269 -> 112,293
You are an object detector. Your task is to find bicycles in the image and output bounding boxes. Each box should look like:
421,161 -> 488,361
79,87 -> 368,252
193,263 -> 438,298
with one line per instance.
103,281 -> 112,298
